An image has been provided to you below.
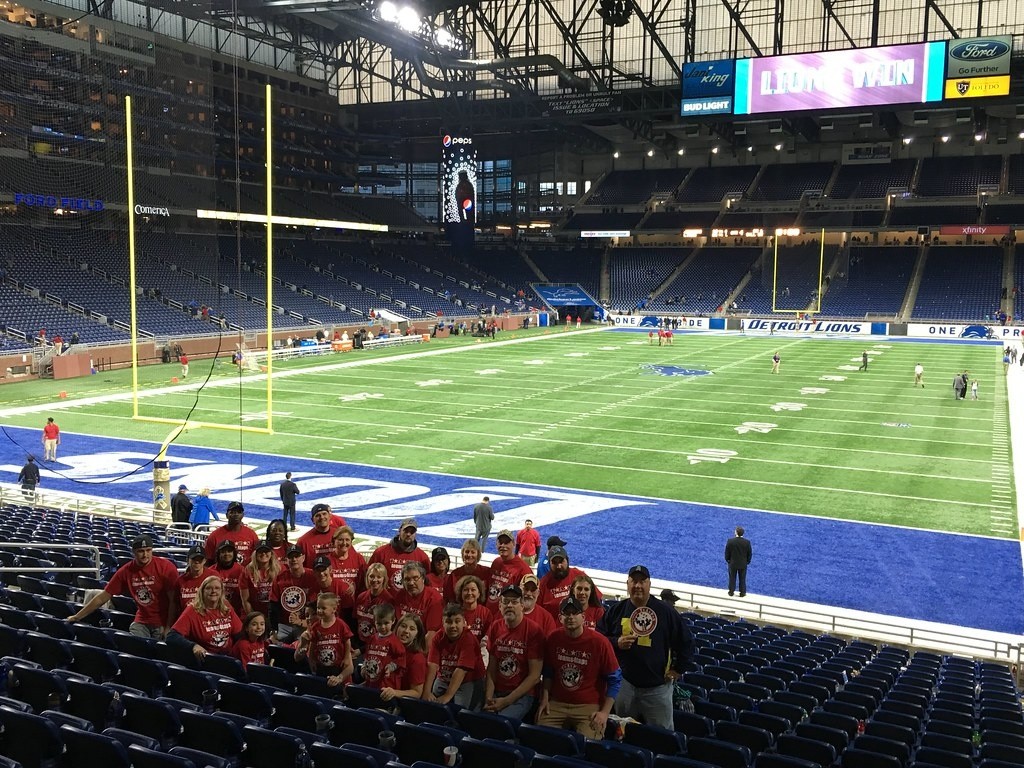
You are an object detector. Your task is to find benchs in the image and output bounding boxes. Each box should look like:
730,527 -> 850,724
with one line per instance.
362,335 -> 425,349
243,344 -> 335,363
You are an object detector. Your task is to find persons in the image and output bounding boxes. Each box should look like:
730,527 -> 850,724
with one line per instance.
0,300 -> 506,384
279,472 -> 301,532
41,417 -> 61,463
912,363 -> 925,388
725,527 -> 752,596
566,314 -> 581,328
858,349 -> 868,372
663,315 -> 678,329
666,291 -> 718,306
611,233 -> 1018,249
771,351 -> 781,374
523,316 -> 529,328
648,328 -> 654,346
450,293 -> 459,302
1000,286 -> 1016,300
170,485 -> 223,545
952,344 -> 1018,401
63,494 -> 698,743
658,327 -> 674,346
438,282 -> 446,293
632,287 -> 655,312
606,309 -> 632,327
18,455 -> 41,507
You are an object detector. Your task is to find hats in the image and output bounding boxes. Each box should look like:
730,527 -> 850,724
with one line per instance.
218,540 -> 233,550
313,555 -> 331,570
432,547 -> 448,560
188,546 -> 205,559
132,535 -> 153,548
226,502 -> 244,512
520,574 -> 538,588
256,540 -> 272,551
547,535 -> 567,550
401,519 -> 417,531
500,585 -> 522,596
286,545 -> 302,555
312,504 -> 329,516
548,546 -> 567,560
660,589 -> 680,602
497,530 -> 514,540
180,485 -> 187,490
559,596 -> 583,610
629,565 -> 651,578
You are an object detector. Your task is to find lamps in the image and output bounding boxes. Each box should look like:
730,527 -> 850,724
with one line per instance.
595,0 -> 634,27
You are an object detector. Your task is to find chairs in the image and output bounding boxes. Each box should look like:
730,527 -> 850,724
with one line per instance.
0,501 -> 1024,768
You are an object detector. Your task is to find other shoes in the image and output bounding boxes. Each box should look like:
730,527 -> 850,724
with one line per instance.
291,526 -> 295,530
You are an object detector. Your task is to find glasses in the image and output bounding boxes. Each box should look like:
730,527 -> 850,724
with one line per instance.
501,599 -> 520,605
564,609 -> 581,615
406,575 -> 422,582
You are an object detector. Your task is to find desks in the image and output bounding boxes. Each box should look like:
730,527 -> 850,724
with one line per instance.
300,340 -> 316,346
331,340 -> 353,353
